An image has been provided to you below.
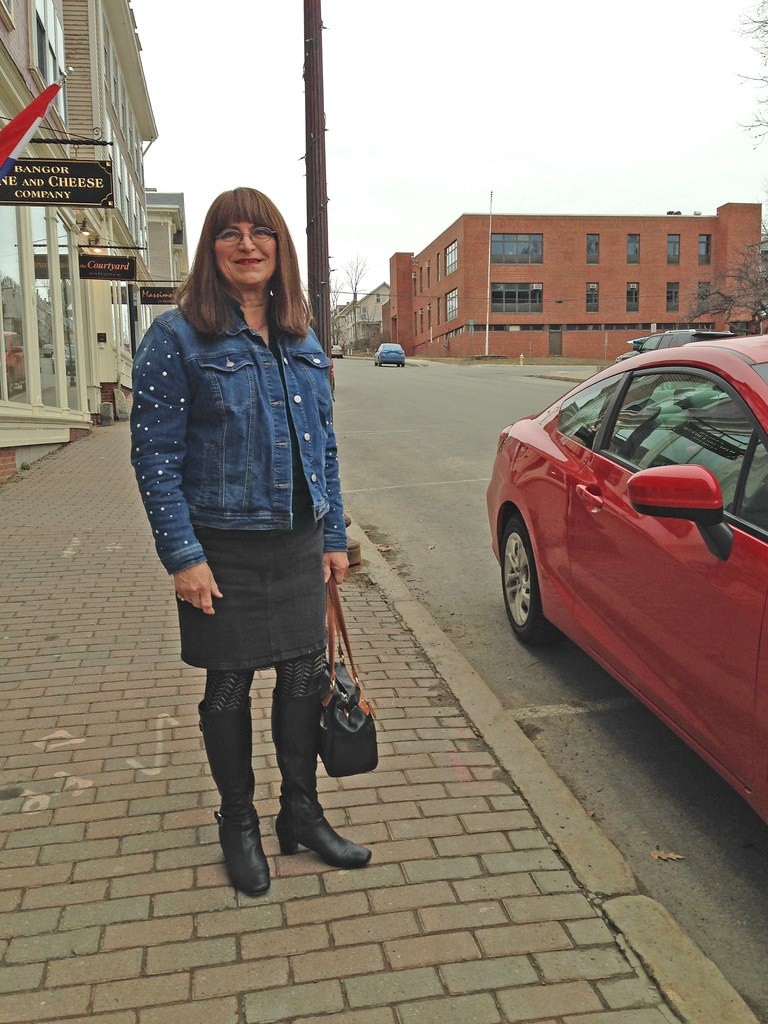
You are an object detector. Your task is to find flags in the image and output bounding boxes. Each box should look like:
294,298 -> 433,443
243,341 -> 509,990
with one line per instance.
1,83 -> 60,179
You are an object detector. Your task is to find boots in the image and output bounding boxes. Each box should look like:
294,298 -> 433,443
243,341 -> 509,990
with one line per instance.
271,687 -> 372,868
197,695 -> 271,896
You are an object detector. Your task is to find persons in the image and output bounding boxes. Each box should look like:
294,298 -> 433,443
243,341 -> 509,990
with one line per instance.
130,186 -> 374,898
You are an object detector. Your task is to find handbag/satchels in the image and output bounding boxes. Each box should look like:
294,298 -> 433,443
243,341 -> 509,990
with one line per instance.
318,571 -> 377,777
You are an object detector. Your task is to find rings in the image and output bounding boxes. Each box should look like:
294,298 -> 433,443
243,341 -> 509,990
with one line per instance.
177,594 -> 183,599
191,601 -> 200,606
181,598 -> 190,601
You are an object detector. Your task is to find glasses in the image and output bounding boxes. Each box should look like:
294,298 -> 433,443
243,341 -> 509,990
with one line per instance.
215,226 -> 276,245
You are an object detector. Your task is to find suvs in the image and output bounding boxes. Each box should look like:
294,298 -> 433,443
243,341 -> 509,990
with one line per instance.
615,329 -> 738,362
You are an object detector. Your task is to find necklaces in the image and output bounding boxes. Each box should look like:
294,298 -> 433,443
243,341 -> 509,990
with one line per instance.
258,322 -> 266,330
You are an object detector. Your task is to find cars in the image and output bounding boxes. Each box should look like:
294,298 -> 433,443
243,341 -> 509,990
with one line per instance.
331,344 -> 344,358
486,335 -> 768,831
374,342 -> 405,367
